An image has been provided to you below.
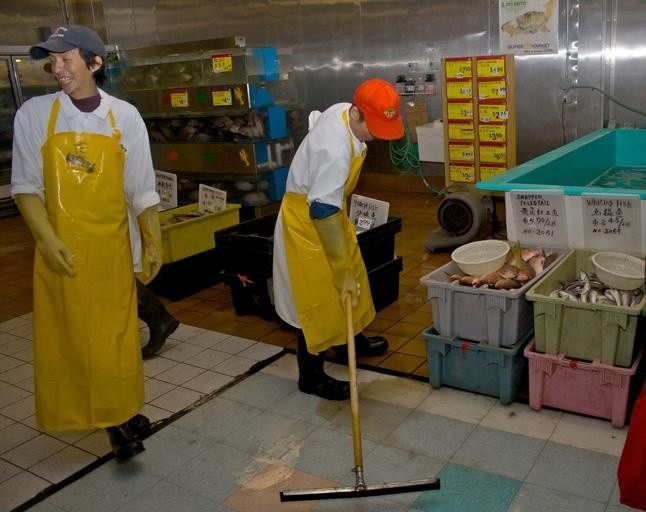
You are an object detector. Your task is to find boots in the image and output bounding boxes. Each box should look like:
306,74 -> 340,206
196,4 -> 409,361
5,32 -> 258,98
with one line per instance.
136,291 -> 179,360
343,331 -> 389,357
105,415 -> 150,465
296,329 -> 351,401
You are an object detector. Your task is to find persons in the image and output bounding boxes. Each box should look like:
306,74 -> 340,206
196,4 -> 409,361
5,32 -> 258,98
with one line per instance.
8,23 -> 164,465
270,79 -> 404,399
41,52 -> 178,364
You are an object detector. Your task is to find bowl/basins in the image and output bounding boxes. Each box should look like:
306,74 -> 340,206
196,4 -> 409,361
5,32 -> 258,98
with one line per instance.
451,238 -> 511,276
590,250 -> 646,293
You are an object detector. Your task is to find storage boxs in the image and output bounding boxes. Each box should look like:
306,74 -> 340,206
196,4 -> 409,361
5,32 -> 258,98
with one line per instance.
539,255 -> 639,362
526,343 -> 639,427
423,329 -> 525,401
423,241 -> 535,338
147,199 -> 403,333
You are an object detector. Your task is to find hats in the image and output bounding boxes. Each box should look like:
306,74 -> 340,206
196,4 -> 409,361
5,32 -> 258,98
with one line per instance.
354,78 -> 405,140
29,25 -> 106,60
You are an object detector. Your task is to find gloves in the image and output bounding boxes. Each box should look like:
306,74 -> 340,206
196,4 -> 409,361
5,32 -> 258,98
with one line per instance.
14,194 -> 78,279
311,211 -> 360,311
135,204 -> 164,286
